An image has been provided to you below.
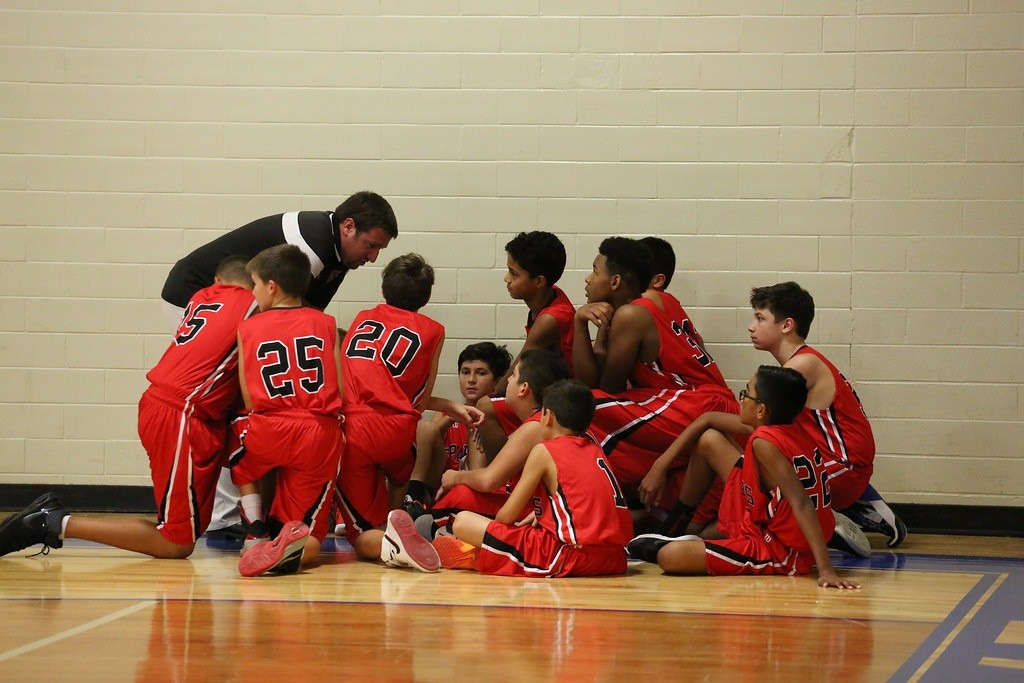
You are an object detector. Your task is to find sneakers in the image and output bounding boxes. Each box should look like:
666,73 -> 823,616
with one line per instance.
334,505 -> 347,536
0,491 -> 70,558
206,523 -> 248,547
380,508 -> 441,572
402,493 -> 428,520
240,520 -> 275,555
635,517 -> 687,536
827,506 -> 870,558
848,483 -> 908,547
626,532 -> 702,564
239,517 -> 312,576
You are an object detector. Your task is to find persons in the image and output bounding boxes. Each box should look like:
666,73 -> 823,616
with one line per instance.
748,282 -> 907,555
160,192 -> 398,549
629,364 -> 862,590
403,342 -> 514,518
431,379 -> 634,577
493,232 -> 741,533
331,253 -> 485,573
228,244 -> 346,576
0,257 -> 261,557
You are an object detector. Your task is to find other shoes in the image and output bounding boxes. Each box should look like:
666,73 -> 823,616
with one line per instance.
429,535 -> 482,572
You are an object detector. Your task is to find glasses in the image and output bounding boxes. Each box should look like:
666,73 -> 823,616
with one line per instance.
738,390 -> 770,414
